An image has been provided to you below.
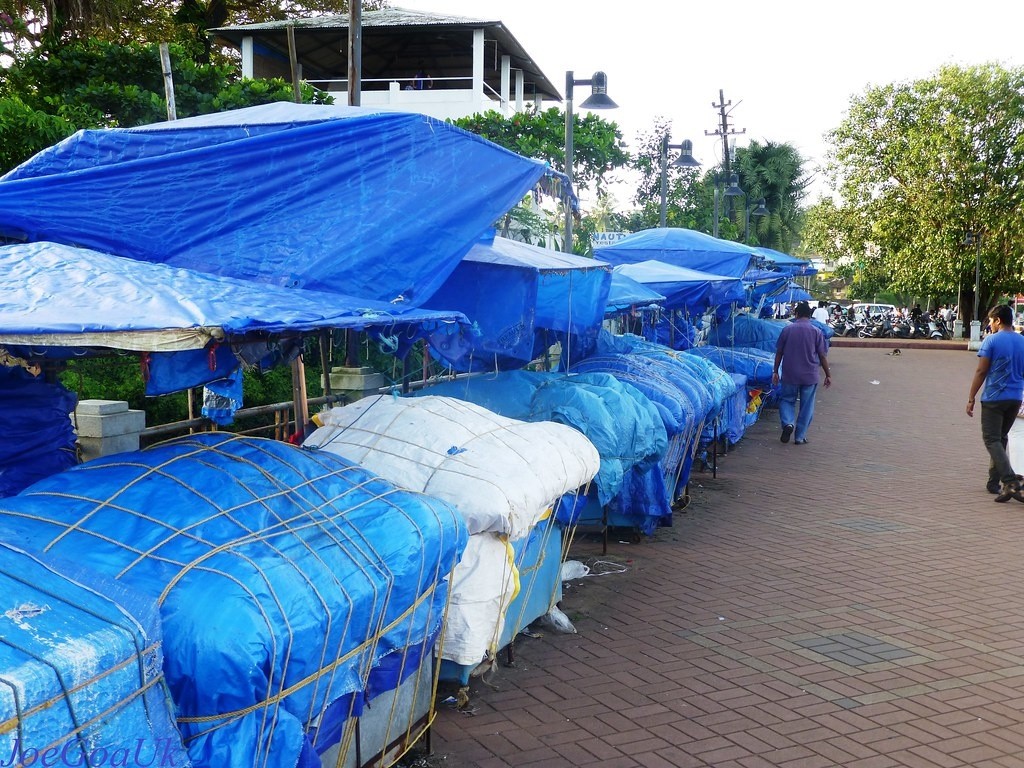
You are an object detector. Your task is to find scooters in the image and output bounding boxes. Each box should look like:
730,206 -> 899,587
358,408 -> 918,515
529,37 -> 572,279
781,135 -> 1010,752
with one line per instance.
826,314 -> 954,340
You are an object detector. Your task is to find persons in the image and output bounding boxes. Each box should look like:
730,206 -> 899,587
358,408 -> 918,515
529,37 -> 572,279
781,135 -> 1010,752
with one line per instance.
812,300 -> 830,324
966,304 -> 1024,502
772,302 -> 833,445
832,302 -> 870,320
896,303 -> 959,325
1008,299 -> 1017,331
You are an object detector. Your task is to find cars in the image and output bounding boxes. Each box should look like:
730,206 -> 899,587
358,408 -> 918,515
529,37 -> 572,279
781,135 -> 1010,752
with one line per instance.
1011,303 -> 1024,329
829,301 -> 839,308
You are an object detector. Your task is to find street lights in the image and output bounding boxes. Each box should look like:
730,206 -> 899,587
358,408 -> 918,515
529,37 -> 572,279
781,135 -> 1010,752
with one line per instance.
659,139 -> 702,229
712,173 -> 746,238
564,68 -> 620,254
742,191 -> 770,239
962,232 -> 982,342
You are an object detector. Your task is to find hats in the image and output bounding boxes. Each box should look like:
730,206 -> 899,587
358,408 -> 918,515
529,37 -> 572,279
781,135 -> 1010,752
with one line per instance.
792,302 -> 817,315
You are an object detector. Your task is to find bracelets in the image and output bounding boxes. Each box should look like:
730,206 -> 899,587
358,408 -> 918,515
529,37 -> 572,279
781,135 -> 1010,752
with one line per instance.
968,398 -> 975,403
772,369 -> 777,373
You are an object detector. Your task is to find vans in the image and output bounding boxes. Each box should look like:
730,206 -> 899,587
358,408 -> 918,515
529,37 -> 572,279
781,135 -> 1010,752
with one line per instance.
847,299 -> 896,321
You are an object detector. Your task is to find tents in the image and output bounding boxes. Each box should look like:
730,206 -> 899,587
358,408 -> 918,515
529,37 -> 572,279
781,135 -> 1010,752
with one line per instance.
1,101 -> 818,440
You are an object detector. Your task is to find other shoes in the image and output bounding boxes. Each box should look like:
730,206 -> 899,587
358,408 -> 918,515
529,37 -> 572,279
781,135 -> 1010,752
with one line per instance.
780,424 -> 794,443
994,480 -> 1023,502
795,438 -> 808,445
986,481 -> 1001,494
